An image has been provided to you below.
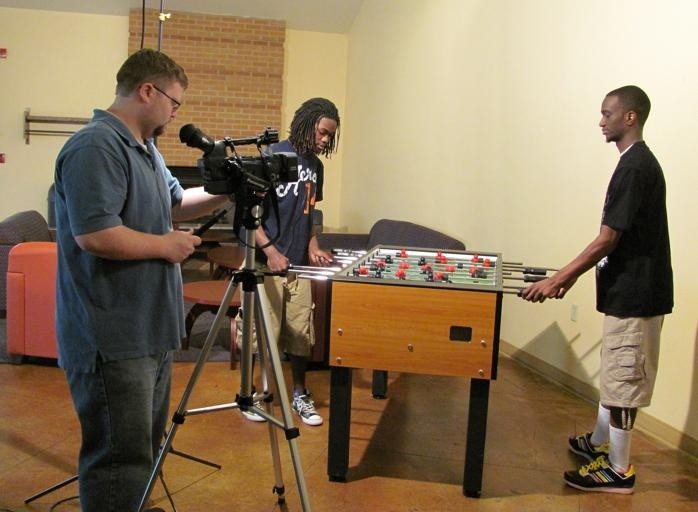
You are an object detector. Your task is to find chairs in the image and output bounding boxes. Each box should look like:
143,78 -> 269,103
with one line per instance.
0,210 -> 60,365
320,220 -> 467,248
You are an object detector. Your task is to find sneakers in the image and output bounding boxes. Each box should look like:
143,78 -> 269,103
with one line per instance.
563,453 -> 636,496
567,431 -> 611,464
237,391 -> 268,423
290,390 -> 325,427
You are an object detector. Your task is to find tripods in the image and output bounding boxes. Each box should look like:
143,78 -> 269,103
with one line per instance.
136,268 -> 312,512
22,302 -> 223,512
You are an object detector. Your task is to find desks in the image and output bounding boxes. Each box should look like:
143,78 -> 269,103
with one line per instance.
180,234 -> 246,371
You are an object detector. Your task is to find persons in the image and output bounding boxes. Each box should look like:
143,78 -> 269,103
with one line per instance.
232,95 -> 345,427
50,48 -> 269,512
519,84 -> 674,495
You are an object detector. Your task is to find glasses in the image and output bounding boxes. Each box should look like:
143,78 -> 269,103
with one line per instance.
138,83 -> 181,112
